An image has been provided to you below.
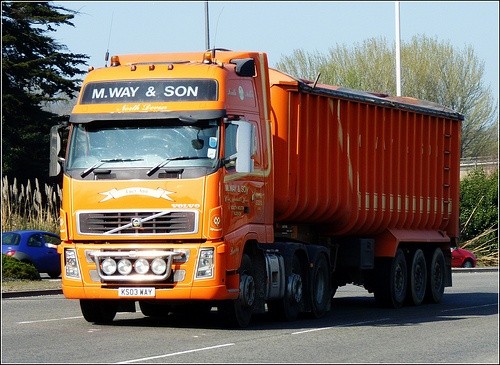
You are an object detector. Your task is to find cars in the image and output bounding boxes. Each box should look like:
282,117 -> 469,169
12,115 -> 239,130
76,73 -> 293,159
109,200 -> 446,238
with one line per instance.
1,230 -> 60,278
450,247 -> 477,268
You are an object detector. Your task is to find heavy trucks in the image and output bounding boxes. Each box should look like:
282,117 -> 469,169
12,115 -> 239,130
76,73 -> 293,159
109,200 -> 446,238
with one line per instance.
49,48 -> 465,325
